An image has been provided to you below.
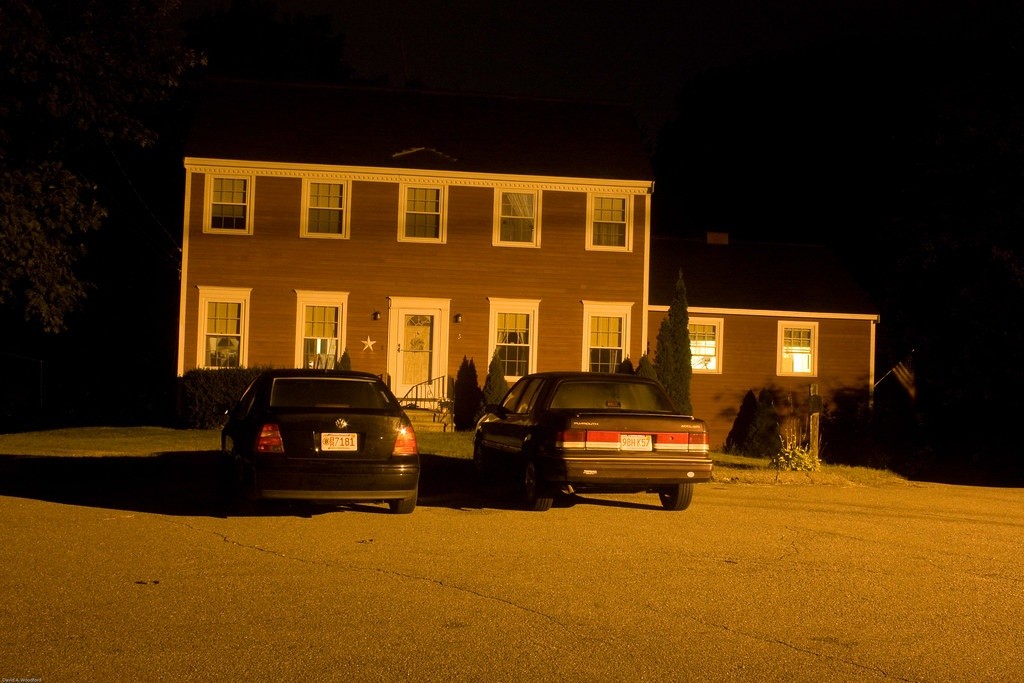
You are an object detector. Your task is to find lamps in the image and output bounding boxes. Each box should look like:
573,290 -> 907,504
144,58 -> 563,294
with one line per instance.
454,313 -> 462,323
373,312 -> 381,320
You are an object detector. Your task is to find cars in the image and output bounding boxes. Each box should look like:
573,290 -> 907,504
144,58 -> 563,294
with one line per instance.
473,371 -> 713,512
212,366 -> 420,516
